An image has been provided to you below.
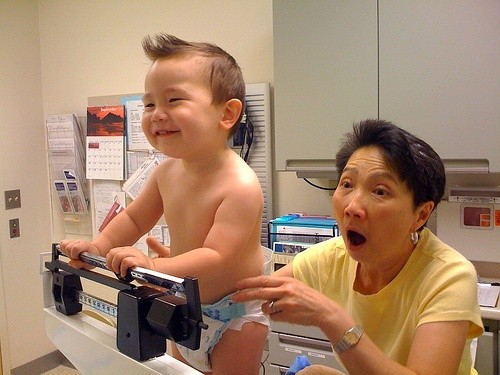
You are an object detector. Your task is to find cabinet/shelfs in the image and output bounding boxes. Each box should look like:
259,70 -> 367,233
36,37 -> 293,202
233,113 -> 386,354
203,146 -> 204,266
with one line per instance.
273,0 -> 500,178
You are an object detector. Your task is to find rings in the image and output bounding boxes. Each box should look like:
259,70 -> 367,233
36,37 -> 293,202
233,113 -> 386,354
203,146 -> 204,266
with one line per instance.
269,301 -> 277,314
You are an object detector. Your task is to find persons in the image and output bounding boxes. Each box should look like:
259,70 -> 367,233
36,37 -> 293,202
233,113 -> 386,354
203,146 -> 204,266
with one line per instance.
145,120 -> 484,375
60,32 -> 271,375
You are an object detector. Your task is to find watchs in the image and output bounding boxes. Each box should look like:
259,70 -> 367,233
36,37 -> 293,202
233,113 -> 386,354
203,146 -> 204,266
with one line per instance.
335,324 -> 364,353
